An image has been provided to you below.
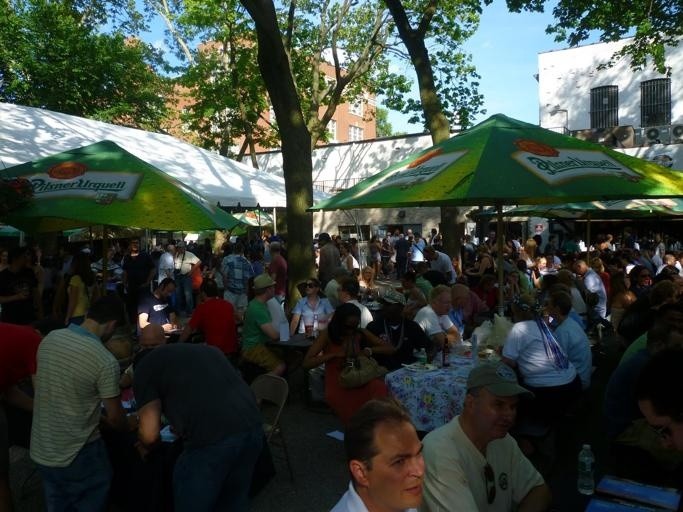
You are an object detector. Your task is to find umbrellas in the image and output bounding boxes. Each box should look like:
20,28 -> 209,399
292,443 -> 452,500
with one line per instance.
305,113 -> 683,317
0,140 -> 240,296
502,198 -> 683,266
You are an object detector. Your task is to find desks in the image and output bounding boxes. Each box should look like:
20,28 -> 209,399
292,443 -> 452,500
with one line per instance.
95,259 -> 624,511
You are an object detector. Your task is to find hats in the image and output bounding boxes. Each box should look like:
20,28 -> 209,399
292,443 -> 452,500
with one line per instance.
268,241 -> 281,250
377,289 -> 407,307
252,273 -> 278,291
465,358 -> 536,403
175,240 -> 187,248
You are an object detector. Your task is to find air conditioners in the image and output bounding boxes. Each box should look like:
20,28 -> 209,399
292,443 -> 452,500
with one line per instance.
639,124 -> 670,148
667,124 -> 681,148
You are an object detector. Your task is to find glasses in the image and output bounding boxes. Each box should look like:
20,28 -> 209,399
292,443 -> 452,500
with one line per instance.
483,463 -> 497,505
647,418 -> 676,441
302,282 -> 317,289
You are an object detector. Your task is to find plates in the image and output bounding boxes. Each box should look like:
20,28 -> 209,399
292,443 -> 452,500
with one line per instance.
406,364 -> 433,372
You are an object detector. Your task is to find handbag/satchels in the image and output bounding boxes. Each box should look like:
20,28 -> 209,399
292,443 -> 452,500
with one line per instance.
338,353 -> 383,393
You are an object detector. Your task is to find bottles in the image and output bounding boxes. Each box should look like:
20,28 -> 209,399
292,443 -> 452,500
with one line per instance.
575,445 -> 596,494
440,337 -> 449,366
416,348 -> 427,372
456,333 -> 480,359
302,313 -> 329,338
